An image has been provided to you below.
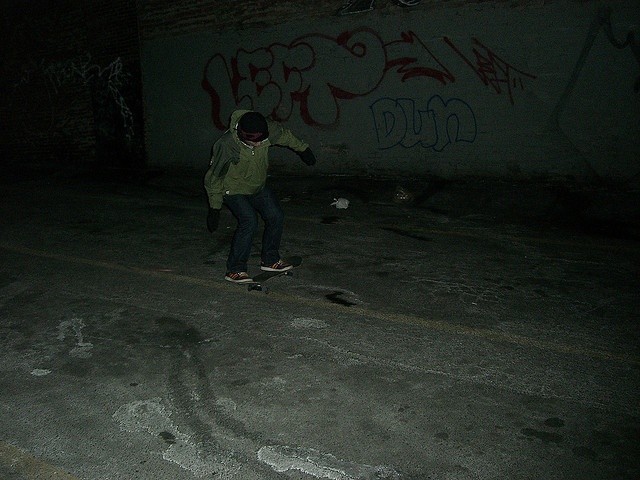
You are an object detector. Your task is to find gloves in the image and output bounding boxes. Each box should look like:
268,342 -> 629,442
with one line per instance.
295,147 -> 317,167
205,206 -> 220,234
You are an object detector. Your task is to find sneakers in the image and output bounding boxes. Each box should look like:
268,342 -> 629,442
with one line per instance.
260,259 -> 294,272
224,272 -> 253,283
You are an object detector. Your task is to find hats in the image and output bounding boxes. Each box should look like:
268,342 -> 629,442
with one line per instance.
237,110 -> 269,142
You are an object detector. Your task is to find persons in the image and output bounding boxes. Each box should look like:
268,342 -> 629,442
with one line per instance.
201,107 -> 317,286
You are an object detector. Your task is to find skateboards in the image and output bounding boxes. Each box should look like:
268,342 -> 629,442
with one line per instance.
238,255 -> 302,292
